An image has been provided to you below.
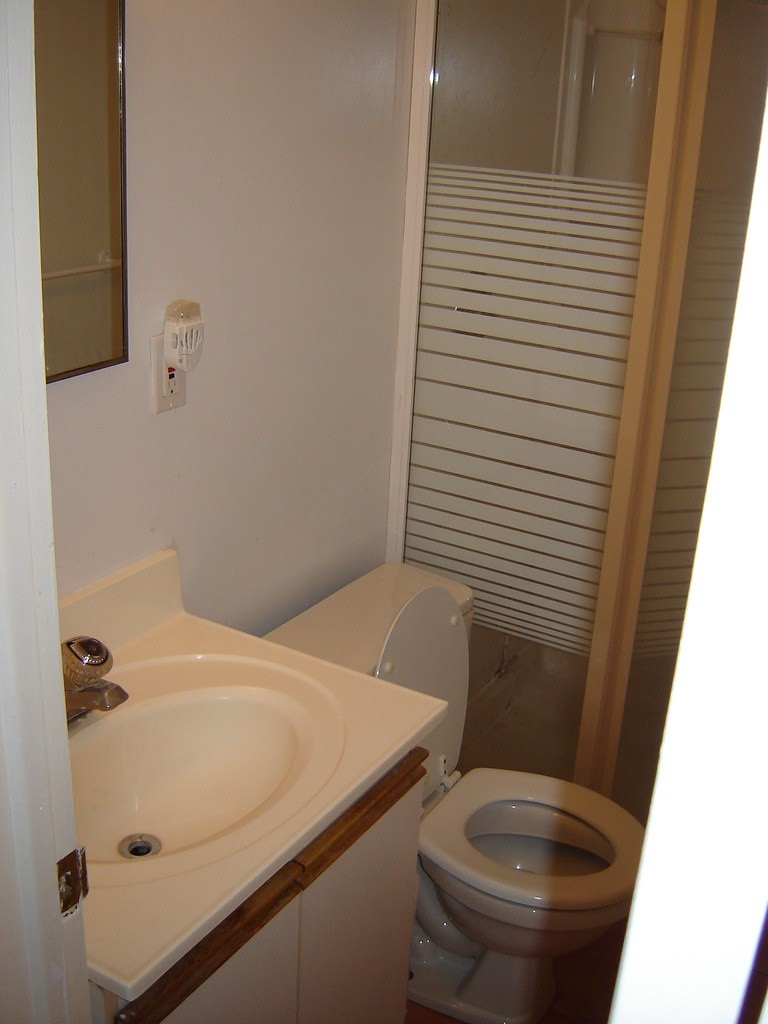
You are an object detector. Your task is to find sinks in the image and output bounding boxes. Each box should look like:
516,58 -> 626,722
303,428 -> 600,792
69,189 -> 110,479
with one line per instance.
71,656 -> 350,893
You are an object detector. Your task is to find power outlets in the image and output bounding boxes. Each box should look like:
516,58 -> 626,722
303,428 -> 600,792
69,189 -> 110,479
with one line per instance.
152,335 -> 187,414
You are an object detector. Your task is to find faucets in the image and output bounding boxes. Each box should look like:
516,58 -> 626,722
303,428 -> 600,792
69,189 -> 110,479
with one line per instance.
64,637 -> 131,726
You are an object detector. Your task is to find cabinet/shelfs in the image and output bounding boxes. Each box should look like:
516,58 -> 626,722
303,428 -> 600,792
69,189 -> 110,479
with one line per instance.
104,746 -> 432,1023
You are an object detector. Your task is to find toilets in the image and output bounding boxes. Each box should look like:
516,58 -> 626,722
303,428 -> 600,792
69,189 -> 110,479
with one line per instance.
253,559 -> 648,1024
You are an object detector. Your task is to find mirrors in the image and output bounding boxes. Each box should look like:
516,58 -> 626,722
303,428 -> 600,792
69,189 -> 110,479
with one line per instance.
33,0 -> 129,383
